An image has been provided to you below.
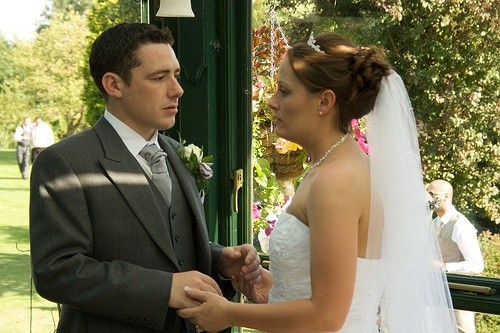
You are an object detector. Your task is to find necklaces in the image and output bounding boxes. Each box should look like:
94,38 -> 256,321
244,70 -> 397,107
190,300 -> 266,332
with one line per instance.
305,132 -> 350,175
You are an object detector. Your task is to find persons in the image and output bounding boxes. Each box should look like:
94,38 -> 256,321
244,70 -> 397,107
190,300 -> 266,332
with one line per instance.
427,180 -> 484,333
15,116 -> 32,178
30,23 -> 262,333
31,117 -> 55,164
177,34 -> 460,333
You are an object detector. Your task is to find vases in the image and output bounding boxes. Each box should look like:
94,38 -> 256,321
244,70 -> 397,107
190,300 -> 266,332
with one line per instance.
258,227 -> 270,255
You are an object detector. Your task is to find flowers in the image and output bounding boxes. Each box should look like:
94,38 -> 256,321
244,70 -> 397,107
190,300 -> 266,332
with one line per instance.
168,129 -> 215,194
253,192 -> 291,237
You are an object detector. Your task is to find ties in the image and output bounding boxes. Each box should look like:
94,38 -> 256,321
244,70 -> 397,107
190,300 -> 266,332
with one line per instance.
436,219 -> 443,236
138,142 -> 172,208
